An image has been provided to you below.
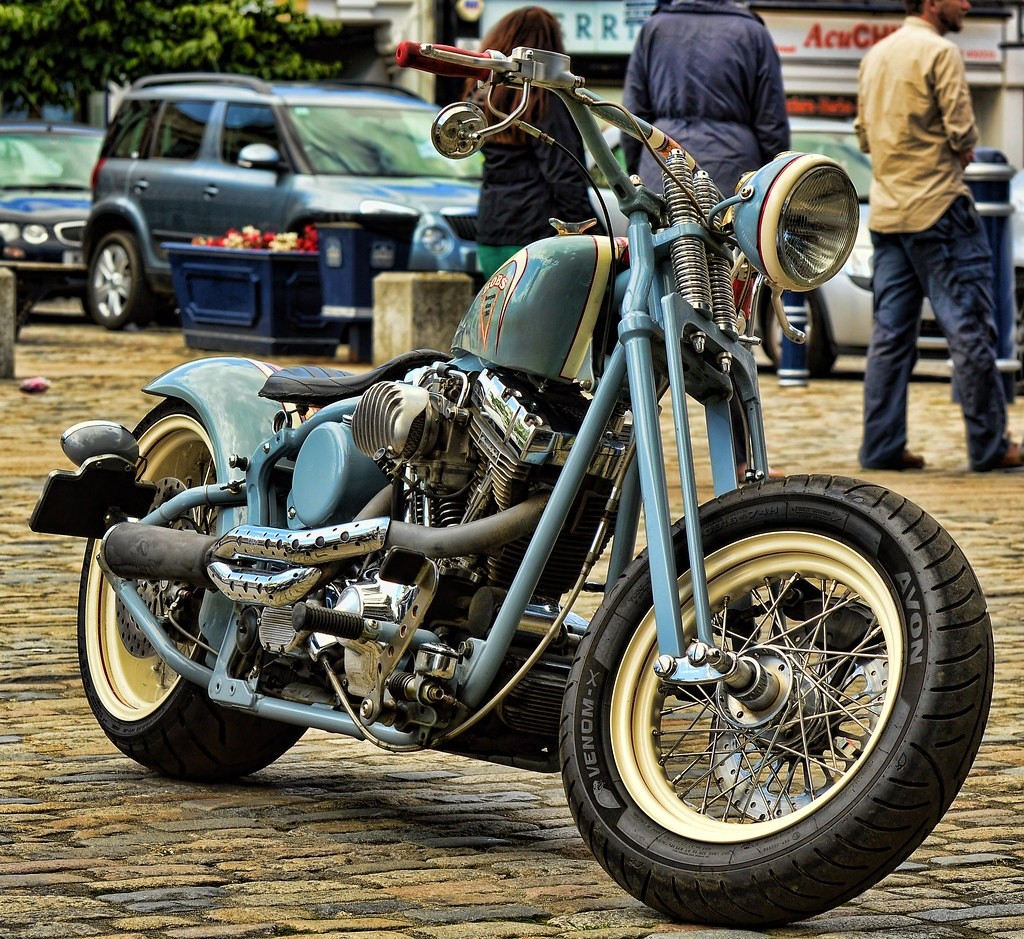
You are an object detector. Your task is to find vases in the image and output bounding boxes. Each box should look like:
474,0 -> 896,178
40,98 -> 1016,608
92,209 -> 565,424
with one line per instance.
161,243 -> 340,356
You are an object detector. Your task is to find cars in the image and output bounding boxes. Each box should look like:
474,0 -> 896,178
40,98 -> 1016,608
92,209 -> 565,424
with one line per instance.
575,112 -> 966,372
0,118 -> 111,319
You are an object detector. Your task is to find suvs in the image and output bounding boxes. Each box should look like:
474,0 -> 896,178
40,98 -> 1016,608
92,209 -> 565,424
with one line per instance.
76,71 -> 483,334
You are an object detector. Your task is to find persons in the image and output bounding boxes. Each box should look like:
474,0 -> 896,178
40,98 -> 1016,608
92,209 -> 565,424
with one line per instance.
463,6 -> 607,280
621,1 -> 790,202
854,0 -> 1024,472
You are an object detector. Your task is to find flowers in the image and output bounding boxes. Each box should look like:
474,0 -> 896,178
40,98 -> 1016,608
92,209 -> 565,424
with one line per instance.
192,225 -> 319,252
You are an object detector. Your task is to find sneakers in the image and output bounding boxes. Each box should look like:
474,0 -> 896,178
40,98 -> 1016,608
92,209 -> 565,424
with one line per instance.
901,450 -> 925,469
1000,433 -> 1024,468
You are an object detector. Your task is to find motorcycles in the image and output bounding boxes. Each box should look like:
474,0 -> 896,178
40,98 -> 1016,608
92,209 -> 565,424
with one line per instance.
26,38 -> 996,928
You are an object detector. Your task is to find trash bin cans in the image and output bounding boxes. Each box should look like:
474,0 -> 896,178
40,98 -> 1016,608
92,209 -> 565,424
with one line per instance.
962,148 -> 1023,407
